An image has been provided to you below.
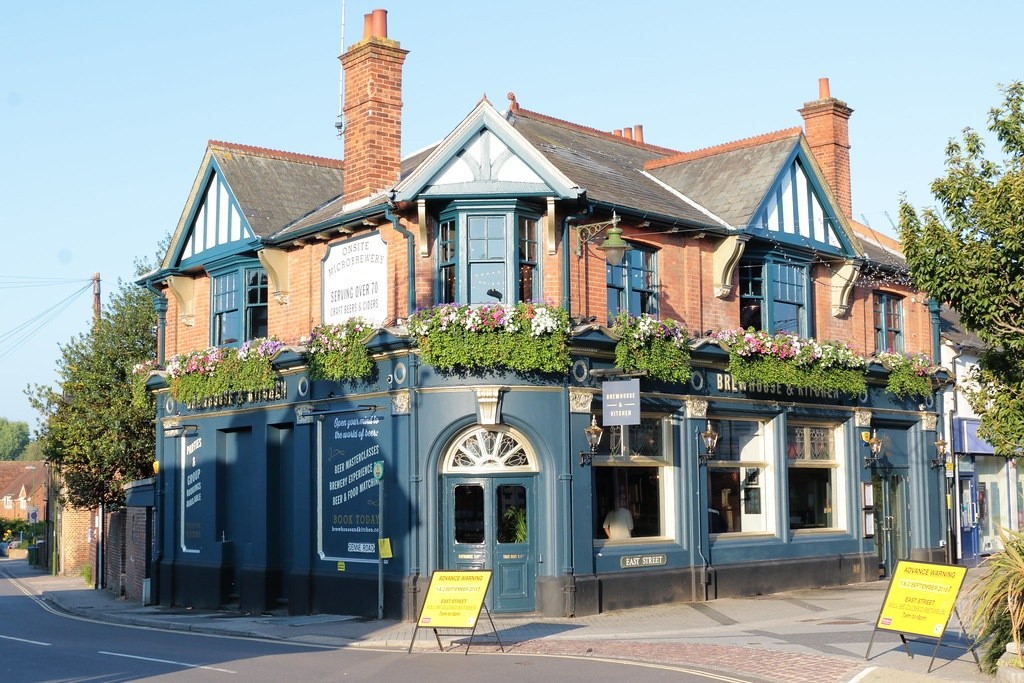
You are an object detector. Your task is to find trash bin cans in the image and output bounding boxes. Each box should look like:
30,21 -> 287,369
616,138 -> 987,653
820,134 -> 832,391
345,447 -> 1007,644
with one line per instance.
28,546 -> 39,565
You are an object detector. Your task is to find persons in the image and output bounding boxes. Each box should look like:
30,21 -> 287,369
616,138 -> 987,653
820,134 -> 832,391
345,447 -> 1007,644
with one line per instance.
603,497 -> 633,539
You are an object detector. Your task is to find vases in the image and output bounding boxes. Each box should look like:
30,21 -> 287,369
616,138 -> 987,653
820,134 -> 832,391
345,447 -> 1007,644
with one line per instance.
406,298 -> 574,375
881,361 -> 893,373
718,340 -> 732,354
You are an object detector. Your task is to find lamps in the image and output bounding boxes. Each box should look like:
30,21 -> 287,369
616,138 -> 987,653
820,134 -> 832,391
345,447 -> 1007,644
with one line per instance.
575,210 -> 631,266
579,415 -> 604,467
931,433 -> 948,469
699,420 -> 719,468
864,429 -> 882,469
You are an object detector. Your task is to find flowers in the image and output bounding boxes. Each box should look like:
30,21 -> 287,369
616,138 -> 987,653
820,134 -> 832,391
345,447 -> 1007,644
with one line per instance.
877,349 -> 935,402
129,334 -> 287,409
305,316 -> 375,382
608,308 -> 693,384
710,326 -> 870,399
